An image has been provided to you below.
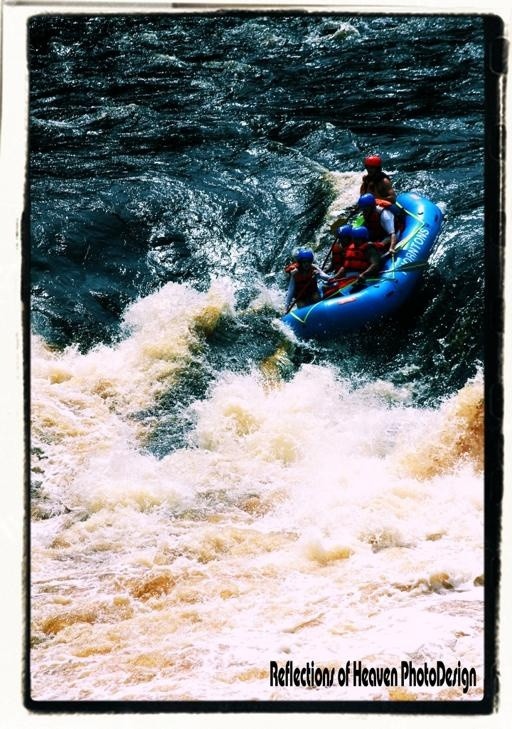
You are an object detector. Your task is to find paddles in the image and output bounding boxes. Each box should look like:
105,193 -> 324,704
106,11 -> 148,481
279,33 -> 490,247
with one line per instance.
378,261 -> 430,277
326,227 -> 421,298
330,205 -> 361,231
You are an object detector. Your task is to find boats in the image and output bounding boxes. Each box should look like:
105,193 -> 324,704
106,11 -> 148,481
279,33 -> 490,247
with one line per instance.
275,190 -> 441,342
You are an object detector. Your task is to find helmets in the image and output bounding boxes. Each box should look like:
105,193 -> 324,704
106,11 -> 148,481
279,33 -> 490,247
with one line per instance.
358,193 -> 374,207
364,156 -> 382,166
297,250 -> 314,263
336,224 -> 352,238
351,226 -> 369,239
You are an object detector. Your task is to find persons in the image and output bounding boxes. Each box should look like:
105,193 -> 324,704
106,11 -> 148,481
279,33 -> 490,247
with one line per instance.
285,251 -> 335,314
318,192 -> 405,298
349,156 -> 397,214
